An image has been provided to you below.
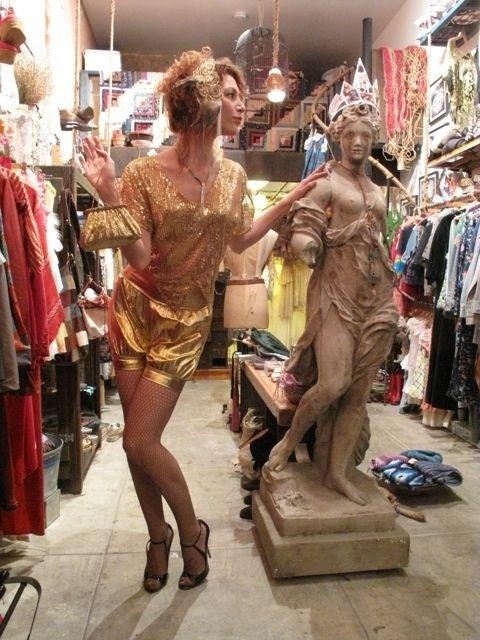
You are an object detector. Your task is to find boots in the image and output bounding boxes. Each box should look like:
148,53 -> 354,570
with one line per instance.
385,372 -> 403,406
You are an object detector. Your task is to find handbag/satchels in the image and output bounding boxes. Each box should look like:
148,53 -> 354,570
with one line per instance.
78,157 -> 142,253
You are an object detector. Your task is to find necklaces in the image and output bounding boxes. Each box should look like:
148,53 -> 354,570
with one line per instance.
173,145 -> 216,207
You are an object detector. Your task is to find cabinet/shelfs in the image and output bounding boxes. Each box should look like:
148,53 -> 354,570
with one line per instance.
35,166 -> 105,498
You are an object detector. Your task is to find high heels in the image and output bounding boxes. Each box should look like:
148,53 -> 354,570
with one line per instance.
177,519 -> 213,590
141,523 -> 174,594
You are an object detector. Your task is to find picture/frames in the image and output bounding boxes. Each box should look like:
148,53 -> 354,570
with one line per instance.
417,172 -> 440,207
103,70 -> 338,156
425,75 -> 449,124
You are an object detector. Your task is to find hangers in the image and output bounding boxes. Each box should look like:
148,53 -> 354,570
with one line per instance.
407,185 -> 480,220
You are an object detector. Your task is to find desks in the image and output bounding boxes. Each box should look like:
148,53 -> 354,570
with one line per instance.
242,356 -> 312,459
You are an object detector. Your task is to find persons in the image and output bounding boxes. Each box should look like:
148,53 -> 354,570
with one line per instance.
266,55 -> 401,507
76,45 -> 328,593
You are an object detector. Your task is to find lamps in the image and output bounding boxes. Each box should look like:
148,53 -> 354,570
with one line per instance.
266,0 -> 286,105
